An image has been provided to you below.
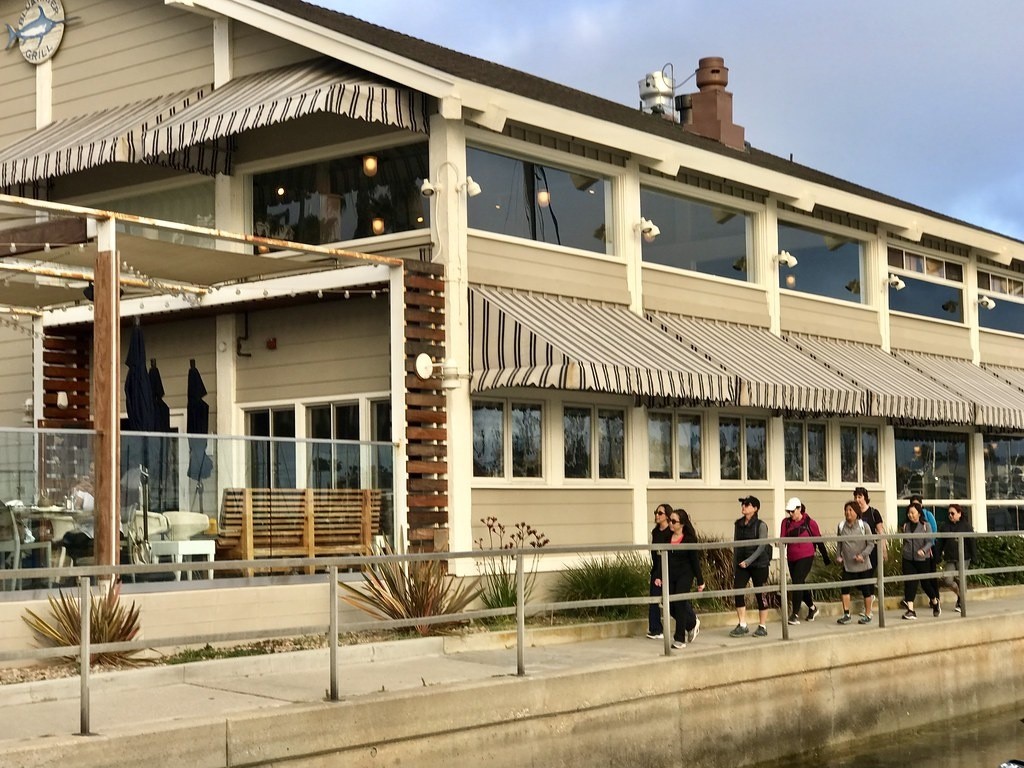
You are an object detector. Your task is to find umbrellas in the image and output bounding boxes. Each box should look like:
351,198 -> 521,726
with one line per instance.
126,322 -> 213,536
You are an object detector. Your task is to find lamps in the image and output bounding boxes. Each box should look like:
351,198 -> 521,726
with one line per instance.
415,353 -> 460,391
942,299 -> 959,312
22,398 -> 33,424
592,225 -> 605,240
634,217 -> 661,239
845,278 -> 859,295
732,255 -> 744,271
457,177 -> 484,197
421,179 -> 443,198
372,217 -> 385,234
538,189 -> 553,209
777,249 -> 798,268
361,154 -> 379,176
882,273 -> 906,292
978,296 -> 997,310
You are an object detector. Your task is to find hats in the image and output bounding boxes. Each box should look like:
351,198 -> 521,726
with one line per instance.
738,495 -> 760,509
785,497 -> 801,511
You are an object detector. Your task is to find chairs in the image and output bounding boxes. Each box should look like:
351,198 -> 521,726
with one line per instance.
69,504 -> 137,583
124,509 -> 209,578
0,505 -> 53,592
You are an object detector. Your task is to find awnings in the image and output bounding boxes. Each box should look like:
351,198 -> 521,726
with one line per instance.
468,282 -> 1024,431
0,55 -> 430,190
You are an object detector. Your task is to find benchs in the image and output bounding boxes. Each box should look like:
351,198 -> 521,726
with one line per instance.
190,487 -> 383,578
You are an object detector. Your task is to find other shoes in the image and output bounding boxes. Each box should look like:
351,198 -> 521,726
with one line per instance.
901,600 -> 908,607
671,640 -> 687,648
752,625 -> 768,637
837,613 -> 851,624
696,615 -> 699,635
788,613 -> 800,625
955,596 -> 962,613
806,605 -> 819,621
858,615 -> 872,624
729,623 -> 749,637
688,618 -> 700,643
933,598 -> 941,617
871,594 -> 876,610
901,609 -> 917,620
858,610 -> 873,617
646,633 -> 664,639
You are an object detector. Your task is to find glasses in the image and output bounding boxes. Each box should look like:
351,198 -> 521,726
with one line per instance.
742,501 -> 752,507
654,510 -> 665,515
787,509 -> 796,513
667,519 -> 680,524
947,512 -> 954,516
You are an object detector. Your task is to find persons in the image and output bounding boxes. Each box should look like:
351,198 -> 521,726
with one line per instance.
646,504 -> 700,639
779,497 -> 832,626
61,460 -> 95,560
654,509 -> 706,650
729,495 -> 770,639
935,504 -> 977,612
835,487 -> 890,626
900,493 -> 941,620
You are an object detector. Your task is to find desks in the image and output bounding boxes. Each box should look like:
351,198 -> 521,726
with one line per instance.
151,540 -> 216,582
347,534 -> 394,571
20,509 -> 80,589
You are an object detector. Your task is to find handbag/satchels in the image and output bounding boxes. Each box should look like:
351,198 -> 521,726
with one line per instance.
745,544 -> 772,568
875,528 -> 889,551
128,527 -> 159,565
